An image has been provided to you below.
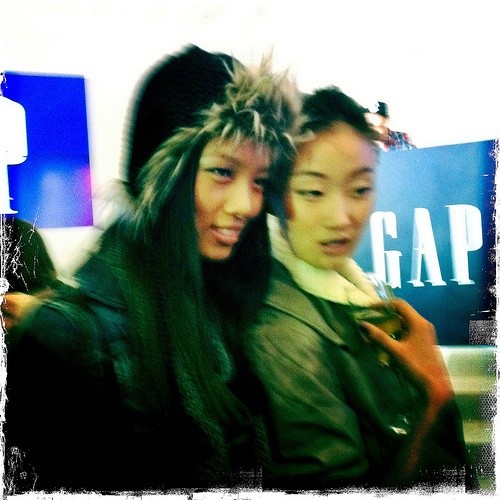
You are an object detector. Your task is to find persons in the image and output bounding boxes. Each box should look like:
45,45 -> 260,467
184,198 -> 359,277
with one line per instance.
2,49 -> 310,497
243,91 -> 474,489
363,104 -> 414,152
2,217 -> 58,329
